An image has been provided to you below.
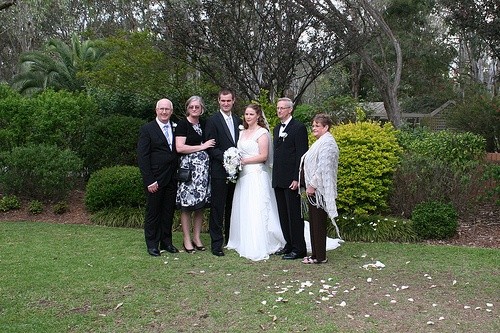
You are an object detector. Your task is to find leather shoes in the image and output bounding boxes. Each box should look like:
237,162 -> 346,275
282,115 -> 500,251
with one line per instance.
147,247 -> 161,256
160,244 -> 178,253
212,245 -> 224,256
275,248 -> 291,255
282,251 -> 307,259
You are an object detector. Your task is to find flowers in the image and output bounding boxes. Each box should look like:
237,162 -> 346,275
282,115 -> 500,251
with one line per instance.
223,146 -> 243,176
239,124 -> 245,130
280,132 -> 288,141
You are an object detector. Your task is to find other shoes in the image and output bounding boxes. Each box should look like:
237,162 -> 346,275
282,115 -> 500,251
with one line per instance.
302,256 -> 328,264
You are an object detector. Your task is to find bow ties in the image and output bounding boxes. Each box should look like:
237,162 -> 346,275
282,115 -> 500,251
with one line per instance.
279,122 -> 286,128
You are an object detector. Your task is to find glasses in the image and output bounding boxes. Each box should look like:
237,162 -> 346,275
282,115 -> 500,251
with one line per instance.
276,105 -> 291,109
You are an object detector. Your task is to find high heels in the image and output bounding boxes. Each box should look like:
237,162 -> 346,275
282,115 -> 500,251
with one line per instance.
191,241 -> 206,251
182,243 -> 196,254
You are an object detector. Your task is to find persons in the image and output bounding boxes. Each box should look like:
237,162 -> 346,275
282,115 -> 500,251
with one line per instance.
173,95 -> 218,255
225,102 -> 287,262
202,88 -> 247,258
269,96 -> 310,262
296,112 -> 342,264
133,98 -> 181,257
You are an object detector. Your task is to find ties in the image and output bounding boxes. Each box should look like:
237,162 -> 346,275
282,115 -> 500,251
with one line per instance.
163,125 -> 170,145
227,117 -> 234,139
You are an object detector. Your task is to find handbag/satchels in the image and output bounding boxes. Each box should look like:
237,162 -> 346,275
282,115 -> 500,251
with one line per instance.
177,167 -> 192,182
